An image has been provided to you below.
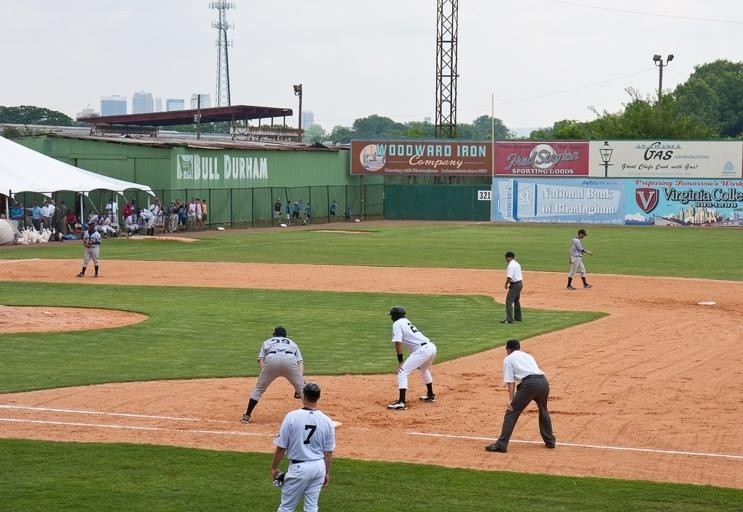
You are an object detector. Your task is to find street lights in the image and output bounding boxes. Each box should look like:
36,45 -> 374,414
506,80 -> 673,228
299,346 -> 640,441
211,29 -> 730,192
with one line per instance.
290,83 -> 304,143
597,141 -> 616,178
653,53 -> 675,121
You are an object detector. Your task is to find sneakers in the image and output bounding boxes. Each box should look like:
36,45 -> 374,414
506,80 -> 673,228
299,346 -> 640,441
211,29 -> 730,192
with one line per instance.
241,415 -> 249,424
485,443 -> 506,453
545,442 -> 555,448
419,394 -> 435,402
387,401 -> 407,410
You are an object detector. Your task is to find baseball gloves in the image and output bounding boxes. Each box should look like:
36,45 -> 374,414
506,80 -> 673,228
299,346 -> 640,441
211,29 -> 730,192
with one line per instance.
83,238 -> 91,248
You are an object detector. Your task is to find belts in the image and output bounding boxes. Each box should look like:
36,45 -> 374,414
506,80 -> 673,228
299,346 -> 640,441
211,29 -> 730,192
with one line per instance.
270,351 -> 292,353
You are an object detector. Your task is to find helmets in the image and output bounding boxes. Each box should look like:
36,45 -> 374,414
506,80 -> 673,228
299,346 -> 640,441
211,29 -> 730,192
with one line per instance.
389,307 -> 406,315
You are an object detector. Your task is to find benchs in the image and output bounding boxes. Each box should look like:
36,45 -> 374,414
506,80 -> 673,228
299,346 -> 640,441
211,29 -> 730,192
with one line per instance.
74,223 -> 119,237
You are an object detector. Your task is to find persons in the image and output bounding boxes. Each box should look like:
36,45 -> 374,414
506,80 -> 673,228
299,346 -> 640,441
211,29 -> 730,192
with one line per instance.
76,223 -> 101,277
242,328 -> 306,424
387,307 -> 436,409
272,383 -> 335,512
9,196 -> 207,240
273,197 -> 353,226
485,340 -> 556,453
500,251 -> 523,324
567,229 -> 593,290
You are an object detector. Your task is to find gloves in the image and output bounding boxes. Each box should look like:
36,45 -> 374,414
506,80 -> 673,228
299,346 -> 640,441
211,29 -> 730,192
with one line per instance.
272,472 -> 285,487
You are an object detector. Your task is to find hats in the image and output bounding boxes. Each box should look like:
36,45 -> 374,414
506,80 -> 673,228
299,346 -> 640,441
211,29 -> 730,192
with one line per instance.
304,383 -> 320,391
272,327 -> 286,335
578,230 -> 587,236
